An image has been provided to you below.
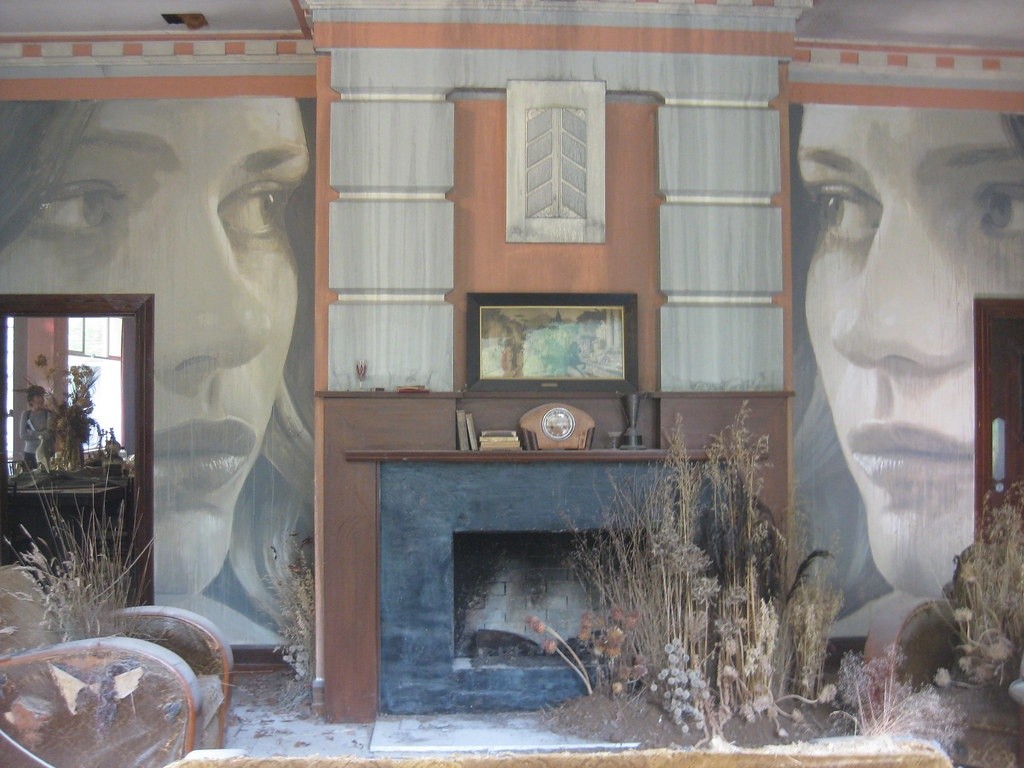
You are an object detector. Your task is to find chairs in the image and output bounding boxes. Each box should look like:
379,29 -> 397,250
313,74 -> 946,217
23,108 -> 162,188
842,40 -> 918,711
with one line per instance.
0,563 -> 237,768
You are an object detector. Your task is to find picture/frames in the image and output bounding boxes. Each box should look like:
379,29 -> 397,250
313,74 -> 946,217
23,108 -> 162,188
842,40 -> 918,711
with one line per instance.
465,292 -> 639,392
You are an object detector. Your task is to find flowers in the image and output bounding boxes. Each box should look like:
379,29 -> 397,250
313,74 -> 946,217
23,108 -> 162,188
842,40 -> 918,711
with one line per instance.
24,352 -> 106,442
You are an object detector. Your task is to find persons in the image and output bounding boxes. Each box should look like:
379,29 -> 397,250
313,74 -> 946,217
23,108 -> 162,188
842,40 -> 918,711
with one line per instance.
0,98 -> 313,643
18,385 -> 53,470
790,103 -> 1023,637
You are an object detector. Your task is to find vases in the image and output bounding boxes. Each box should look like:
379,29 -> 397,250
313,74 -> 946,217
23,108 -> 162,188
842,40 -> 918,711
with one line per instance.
61,434 -> 85,477
